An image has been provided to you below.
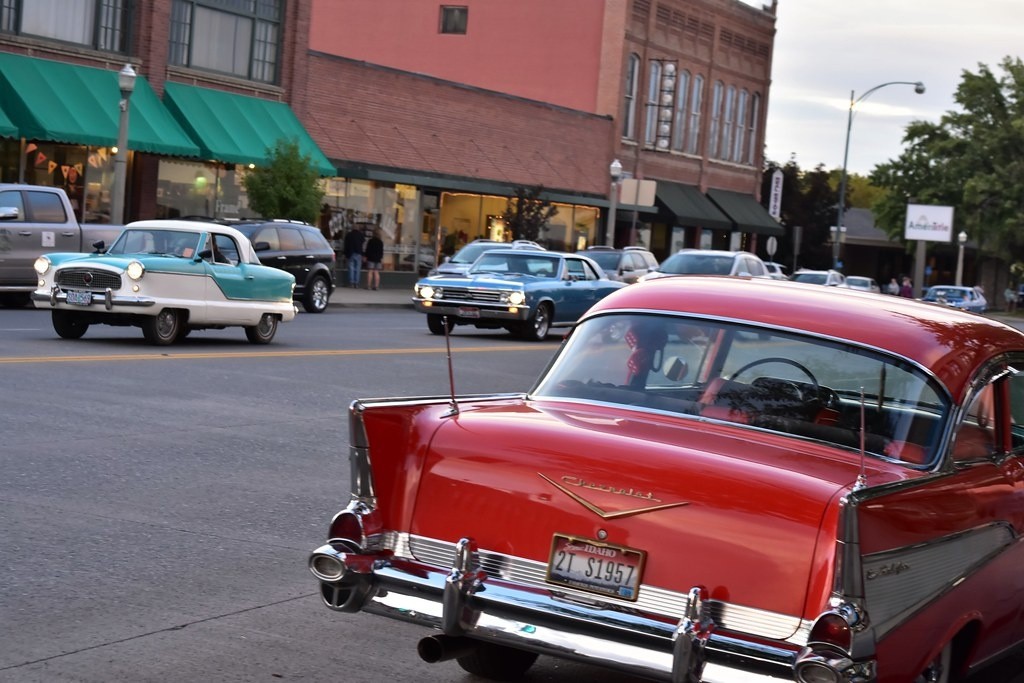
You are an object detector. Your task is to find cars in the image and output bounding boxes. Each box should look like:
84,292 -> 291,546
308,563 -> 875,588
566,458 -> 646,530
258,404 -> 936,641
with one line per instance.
916,286 -> 988,314
29,218 -> 299,346
305,273 -> 1023,681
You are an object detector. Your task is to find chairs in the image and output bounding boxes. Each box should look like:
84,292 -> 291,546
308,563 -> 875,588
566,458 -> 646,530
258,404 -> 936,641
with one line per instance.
698,378 -> 839,429
948,421 -> 998,463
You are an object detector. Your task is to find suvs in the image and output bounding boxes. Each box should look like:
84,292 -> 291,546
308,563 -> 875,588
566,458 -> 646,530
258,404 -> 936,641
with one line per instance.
170,218 -> 337,314
410,239 -> 882,345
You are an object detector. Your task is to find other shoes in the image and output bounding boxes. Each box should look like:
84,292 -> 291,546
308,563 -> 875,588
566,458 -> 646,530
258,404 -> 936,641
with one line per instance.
351,284 -> 359,289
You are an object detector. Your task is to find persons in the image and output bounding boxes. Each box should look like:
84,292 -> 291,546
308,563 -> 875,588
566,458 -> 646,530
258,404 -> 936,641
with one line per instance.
206,235 -> 230,263
1004,281 -> 1024,313
888,277 -> 913,298
344,223 -> 384,290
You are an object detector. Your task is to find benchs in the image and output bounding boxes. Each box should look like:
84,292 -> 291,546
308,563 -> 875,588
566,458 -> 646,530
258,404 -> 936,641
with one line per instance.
555,384 -> 926,465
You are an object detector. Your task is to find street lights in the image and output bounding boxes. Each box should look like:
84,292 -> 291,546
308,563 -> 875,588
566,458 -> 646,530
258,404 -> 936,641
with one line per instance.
954,228 -> 968,294
110,62 -> 137,225
605,157 -> 622,250
823,80 -> 926,262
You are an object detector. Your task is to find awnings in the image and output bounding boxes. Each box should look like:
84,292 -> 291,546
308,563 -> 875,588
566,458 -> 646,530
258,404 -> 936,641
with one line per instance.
639,177 -> 785,235
0,51 -> 338,178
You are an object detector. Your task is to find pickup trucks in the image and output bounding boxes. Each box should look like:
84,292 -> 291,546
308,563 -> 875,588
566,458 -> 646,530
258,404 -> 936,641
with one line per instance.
0,184 -> 145,308
